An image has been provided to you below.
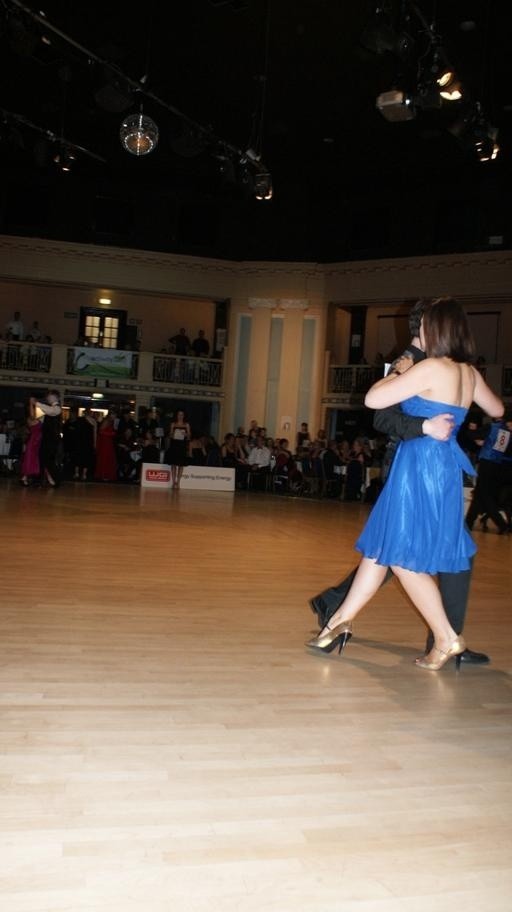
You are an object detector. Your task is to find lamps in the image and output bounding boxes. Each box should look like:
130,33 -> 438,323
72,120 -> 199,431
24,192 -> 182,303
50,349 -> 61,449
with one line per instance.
225,144 -> 274,202
373,1 -> 498,164
120,77 -> 161,157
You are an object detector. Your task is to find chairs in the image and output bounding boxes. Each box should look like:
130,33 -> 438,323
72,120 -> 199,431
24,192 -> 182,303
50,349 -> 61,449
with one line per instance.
234,447 -> 381,504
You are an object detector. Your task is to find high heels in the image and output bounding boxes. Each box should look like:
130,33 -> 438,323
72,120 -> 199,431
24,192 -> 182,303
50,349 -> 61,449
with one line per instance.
480,518 -> 488,530
304,621 -> 353,657
415,634 -> 466,672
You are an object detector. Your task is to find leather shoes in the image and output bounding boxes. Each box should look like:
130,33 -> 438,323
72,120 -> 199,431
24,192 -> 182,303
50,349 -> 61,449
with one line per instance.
423,645 -> 490,666
311,596 -> 332,628
